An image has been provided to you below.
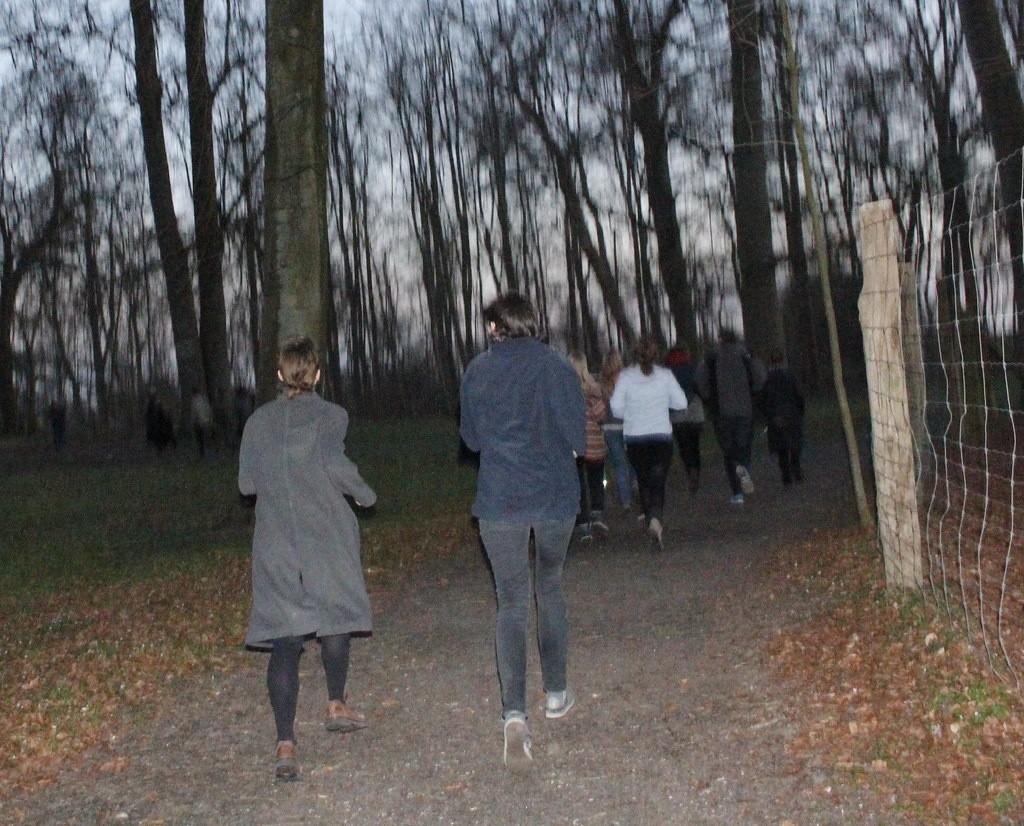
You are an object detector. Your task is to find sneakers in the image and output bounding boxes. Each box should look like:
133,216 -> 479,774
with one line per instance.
276,741 -> 297,784
542,688 -> 576,718
504,711 -> 533,778
326,699 -> 367,733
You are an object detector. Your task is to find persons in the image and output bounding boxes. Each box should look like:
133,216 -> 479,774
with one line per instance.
458,292 -> 587,776
235,339 -> 378,785
565,323 -> 806,552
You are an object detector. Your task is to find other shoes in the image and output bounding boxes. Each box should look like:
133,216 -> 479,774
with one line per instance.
729,494 -> 743,503
795,467 -> 801,480
638,514 -> 647,526
783,479 -> 792,487
632,482 -> 639,490
623,503 -> 631,513
580,522 -> 593,545
689,470 -> 698,494
736,464 -> 754,493
591,512 -> 610,535
647,518 -> 664,552
